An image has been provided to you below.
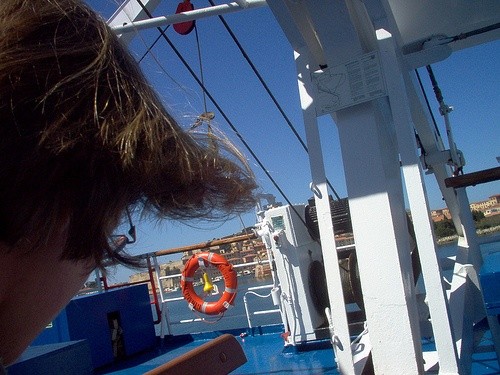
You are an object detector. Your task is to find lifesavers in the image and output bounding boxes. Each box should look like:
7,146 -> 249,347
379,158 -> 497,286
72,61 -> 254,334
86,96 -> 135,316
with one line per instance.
179,251 -> 238,316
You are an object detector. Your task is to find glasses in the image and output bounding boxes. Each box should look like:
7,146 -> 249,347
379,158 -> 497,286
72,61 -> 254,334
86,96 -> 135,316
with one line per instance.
101,207 -> 136,258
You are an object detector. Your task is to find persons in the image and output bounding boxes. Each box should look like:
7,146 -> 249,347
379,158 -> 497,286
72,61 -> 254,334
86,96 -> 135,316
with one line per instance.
0,0 -> 257,368
